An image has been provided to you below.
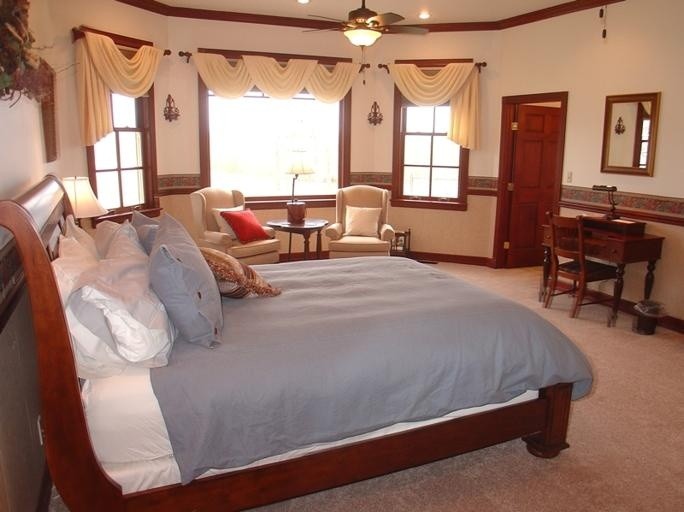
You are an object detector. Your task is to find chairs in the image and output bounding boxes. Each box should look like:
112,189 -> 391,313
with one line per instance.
326,184 -> 395,259
190,186 -> 280,264
544,210 -> 624,327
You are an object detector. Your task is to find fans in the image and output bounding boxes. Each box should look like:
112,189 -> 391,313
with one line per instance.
301,0 -> 430,36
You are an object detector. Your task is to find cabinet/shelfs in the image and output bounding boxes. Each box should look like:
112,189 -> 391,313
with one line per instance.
390,229 -> 413,258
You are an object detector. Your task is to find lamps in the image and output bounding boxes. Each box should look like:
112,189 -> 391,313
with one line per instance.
344,28 -> 382,85
367,102 -> 384,125
615,116 -> 625,135
592,185 -> 618,220
61,176 -> 109,227
284,150 -> 315,225
164,95 -> 180,122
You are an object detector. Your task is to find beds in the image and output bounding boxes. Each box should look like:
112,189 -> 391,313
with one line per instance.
0,174 -> 593,510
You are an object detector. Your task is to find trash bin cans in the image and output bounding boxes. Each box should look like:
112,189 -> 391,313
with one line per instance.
636,300 -> 661,335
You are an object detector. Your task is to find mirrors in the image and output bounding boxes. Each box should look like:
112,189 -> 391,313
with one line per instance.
600,91 -> 661,177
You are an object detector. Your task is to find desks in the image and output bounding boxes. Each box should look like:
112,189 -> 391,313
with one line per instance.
538,219 -> 665,327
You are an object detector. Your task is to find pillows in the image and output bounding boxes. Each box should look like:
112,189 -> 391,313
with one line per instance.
343,204 -> 383,237
